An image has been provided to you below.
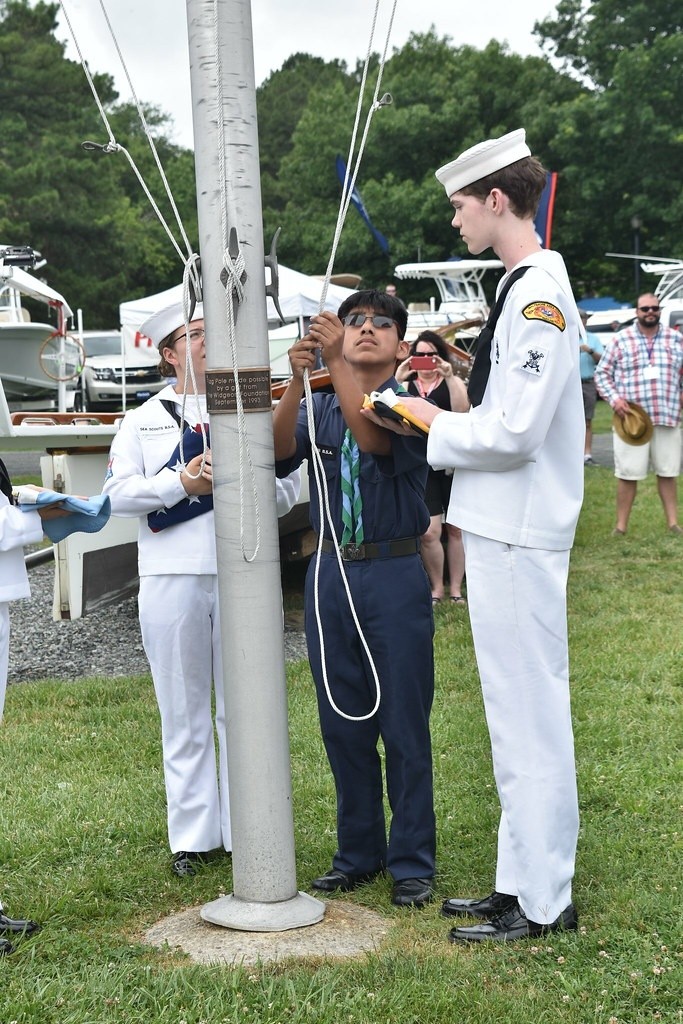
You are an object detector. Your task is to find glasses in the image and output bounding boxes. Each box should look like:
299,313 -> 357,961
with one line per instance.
341,313 -> 397,328
639,307 -> 659,312
169,328 -> 205,345
412,352 -> 438,357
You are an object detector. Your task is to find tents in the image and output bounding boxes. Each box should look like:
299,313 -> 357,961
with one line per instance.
120,258 -> 361,415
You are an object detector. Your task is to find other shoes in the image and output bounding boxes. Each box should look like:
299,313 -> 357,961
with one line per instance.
172,851 -> 204,878
585,458 -> 601,466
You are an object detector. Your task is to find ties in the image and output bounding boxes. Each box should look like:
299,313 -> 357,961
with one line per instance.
468,266 -> 531,408
342,382 -> 406,547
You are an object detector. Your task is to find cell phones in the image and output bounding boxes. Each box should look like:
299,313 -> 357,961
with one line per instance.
411,357 -> 438,370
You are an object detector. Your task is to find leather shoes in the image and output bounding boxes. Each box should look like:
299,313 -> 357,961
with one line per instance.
0,940 -> 14,956
0,911 -> 38,934
442,890 -> 517,919
392,878 -> 435,907
449,901 -> 578,945
312,869 -> 384,893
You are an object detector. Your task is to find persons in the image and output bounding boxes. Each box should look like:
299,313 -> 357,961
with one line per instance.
0,461 -> 90,957
578,309 -> 605,464
386,286 -> 406,307
593,293 -> 683,535
359,128 -> 586,945
394,331 -> 470,606
272,289 -> 441,907
612,320 -> 625,332
101,299 -> 300,876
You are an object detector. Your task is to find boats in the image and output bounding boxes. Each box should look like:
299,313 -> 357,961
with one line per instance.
0,243 -> 82,398
0,253 -> 683,623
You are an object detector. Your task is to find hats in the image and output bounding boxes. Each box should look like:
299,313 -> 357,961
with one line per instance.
613,402 -> 653,445
578,308 -> 592,319
139,298 -> 204,348
435,128 -> 532,197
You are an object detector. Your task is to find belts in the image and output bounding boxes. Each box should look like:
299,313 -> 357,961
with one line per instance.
320,538 -> 421,562
582,378 -> 594,383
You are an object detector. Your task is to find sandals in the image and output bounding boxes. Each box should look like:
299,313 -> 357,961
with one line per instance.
431,598 -> 439,606
449,597 -> 466,605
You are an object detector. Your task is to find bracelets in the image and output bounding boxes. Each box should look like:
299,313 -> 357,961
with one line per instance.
588,348 -> 594,354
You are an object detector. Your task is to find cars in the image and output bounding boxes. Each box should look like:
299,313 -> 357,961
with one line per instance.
66,328 -> 166,415
